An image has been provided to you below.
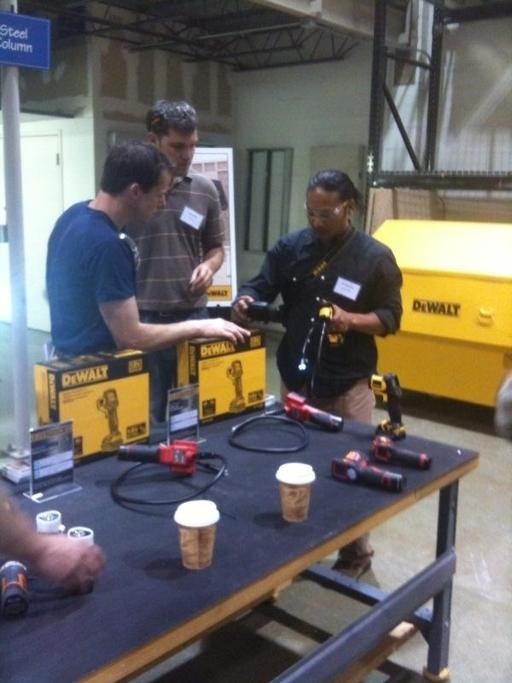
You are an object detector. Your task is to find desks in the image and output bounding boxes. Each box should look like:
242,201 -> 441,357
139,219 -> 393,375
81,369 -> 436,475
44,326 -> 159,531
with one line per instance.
0,403 -> 479,683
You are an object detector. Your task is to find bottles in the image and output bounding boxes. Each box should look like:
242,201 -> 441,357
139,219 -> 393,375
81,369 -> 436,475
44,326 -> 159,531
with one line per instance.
34,508 -> 94,548
0,559 -> 29,616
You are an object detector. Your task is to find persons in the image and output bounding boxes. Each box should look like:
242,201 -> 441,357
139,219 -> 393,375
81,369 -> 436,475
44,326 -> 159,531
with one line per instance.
48,136 -> 253,353
226,166 -> 405,587
1,483 -> 110,603
122,97 -> 228,431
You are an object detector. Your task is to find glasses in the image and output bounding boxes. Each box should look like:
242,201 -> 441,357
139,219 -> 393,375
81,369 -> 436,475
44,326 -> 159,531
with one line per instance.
301,195 -> 350,219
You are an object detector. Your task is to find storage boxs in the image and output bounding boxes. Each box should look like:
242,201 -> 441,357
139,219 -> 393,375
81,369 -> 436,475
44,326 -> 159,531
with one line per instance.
27,323 -> 271,468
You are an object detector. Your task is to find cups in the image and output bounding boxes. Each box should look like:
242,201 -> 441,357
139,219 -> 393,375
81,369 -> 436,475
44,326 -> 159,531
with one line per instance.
274,462 -> 317,523
173,500 -> 220,570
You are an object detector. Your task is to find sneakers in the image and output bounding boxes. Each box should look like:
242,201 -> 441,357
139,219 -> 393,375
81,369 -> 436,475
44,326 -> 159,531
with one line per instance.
332,543 -> 372,576
